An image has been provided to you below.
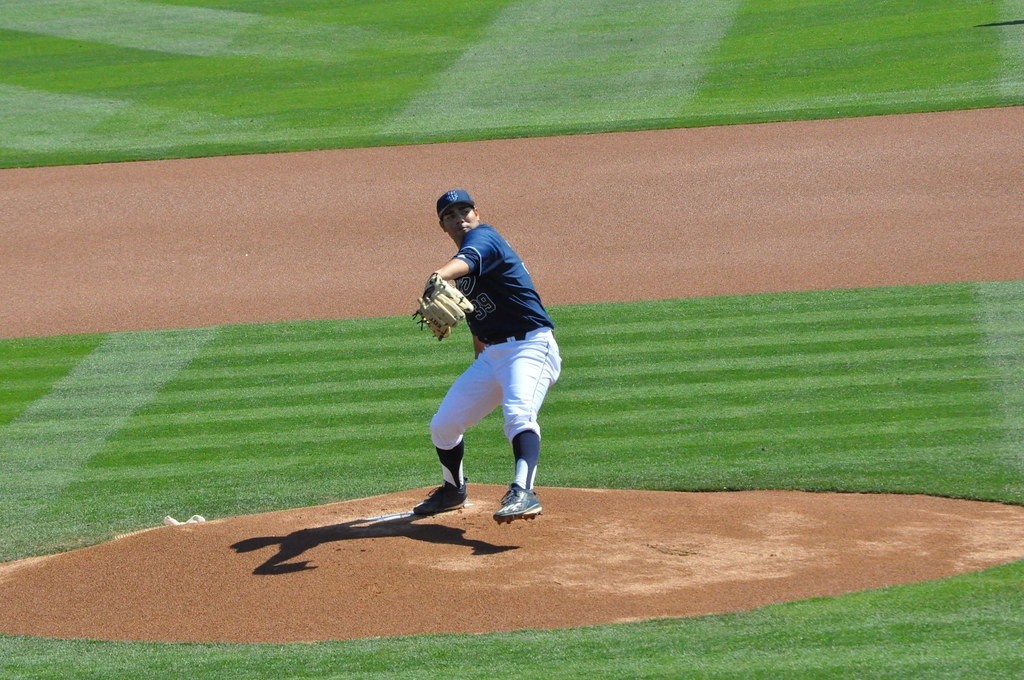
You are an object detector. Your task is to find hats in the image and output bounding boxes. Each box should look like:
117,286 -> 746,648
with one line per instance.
436,190 -> 475,220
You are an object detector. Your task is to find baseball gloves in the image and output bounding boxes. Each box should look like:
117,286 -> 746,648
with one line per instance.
416,280 -> 476,340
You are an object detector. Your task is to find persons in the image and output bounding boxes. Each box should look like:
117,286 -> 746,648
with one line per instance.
413,189 -> 562,526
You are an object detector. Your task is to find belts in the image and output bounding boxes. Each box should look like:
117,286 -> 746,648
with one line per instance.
486,332 -> 524,346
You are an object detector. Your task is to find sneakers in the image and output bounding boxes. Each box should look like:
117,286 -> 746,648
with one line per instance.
494,483 -> 542,524
412,484 -> 468,516
